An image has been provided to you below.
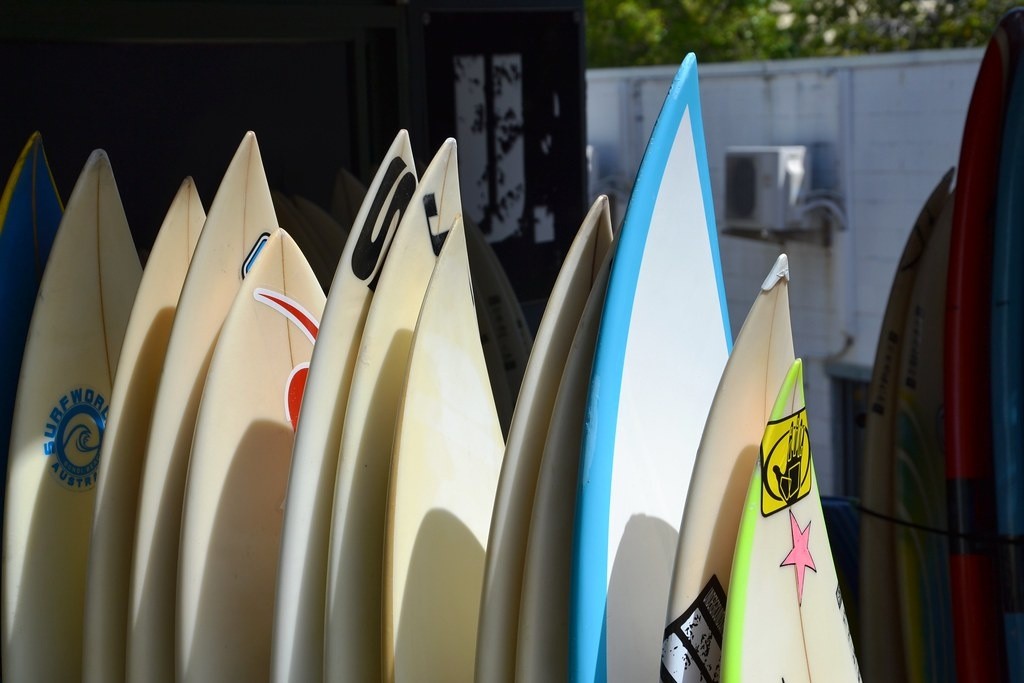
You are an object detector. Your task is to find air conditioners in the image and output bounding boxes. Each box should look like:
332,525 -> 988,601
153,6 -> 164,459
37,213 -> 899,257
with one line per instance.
724,143 -> 850,231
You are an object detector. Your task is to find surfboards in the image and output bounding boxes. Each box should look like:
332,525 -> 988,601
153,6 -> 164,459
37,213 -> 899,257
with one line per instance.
0,50 -> 864,683
859,10 -> 1024,683
